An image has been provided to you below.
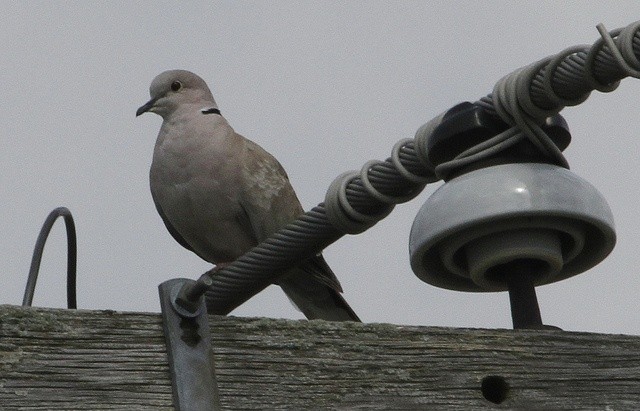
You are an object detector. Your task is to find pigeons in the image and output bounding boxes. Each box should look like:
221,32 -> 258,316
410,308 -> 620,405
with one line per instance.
134,69 -> 361,320
